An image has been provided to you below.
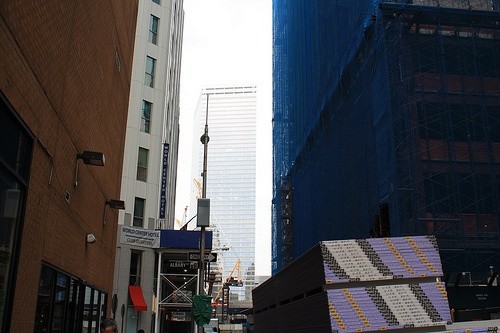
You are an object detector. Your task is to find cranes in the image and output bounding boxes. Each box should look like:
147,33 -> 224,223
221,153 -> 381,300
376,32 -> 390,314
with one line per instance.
191,178 -> 206,199
213,257 -> 241,322
176,205 -> 191,231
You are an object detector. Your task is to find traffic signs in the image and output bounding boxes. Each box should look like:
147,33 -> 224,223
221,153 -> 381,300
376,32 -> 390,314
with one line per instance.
187,252 -> 218,264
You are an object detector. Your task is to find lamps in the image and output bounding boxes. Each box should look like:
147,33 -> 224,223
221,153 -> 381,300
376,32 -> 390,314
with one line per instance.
105,199 -> 126,210
76,151 -> 106,166
